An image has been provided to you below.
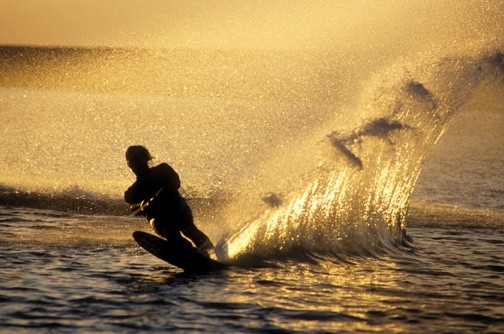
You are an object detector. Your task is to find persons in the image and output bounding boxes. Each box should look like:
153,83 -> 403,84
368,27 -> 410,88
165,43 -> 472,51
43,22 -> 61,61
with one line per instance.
124,146 -> 217,260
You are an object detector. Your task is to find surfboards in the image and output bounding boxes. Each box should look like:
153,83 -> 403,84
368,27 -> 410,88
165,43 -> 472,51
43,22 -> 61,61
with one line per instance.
132,230 -> 223,271
